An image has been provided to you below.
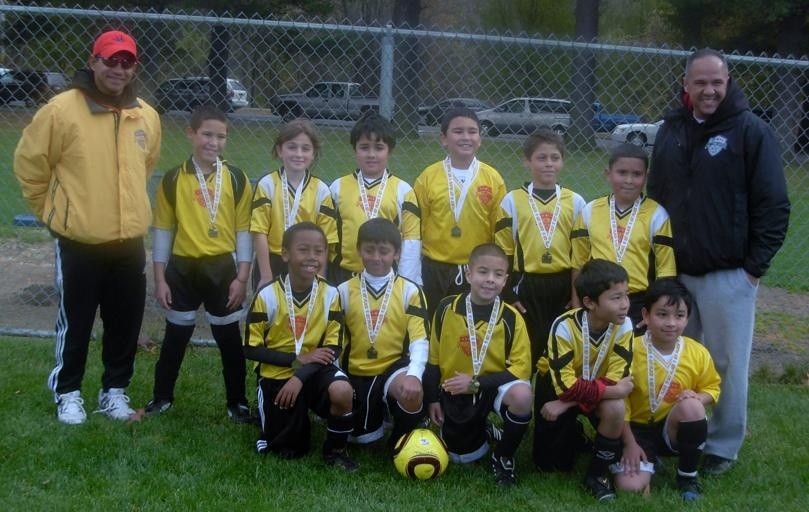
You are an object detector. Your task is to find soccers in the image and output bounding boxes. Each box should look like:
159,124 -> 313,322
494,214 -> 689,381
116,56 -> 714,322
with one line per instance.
392,429 -> 449,480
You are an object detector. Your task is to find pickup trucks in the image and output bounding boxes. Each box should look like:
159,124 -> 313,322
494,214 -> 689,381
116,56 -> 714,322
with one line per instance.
269,81 -> 396,124
593,102 -> 641,134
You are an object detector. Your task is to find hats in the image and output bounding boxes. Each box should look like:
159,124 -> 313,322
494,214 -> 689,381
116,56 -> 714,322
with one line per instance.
92,28 -> 139,61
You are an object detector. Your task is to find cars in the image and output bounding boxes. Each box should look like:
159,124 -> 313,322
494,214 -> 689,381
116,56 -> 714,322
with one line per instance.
612,118 -> 666,147
416,97 -> 494,126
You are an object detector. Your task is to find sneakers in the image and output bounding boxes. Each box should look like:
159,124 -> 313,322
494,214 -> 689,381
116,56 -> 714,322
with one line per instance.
702,438 -> 739,474
678,467 -> 705,504
490,451 -> 518,486
483,417 -> 504,440
51,388 -> 88,425
581,472 -> 619,505
573,417 -> 592,449
225,398 -> 249,426
96,385 -> 136,425
144,391 -> 177,419
321,446 -> 358,476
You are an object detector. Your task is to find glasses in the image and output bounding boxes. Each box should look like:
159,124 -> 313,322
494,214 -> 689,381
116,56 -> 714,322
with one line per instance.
93,54 -> 136,69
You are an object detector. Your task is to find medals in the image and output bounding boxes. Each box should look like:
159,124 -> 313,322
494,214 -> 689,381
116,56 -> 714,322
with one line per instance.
290,358 -> 304,370
540,250 -> 552,263
207,226 -> 218,238
647,415 -> 654,424
450,224 -> 461,237
468,378 -> 481,394
366,345 -> 378,359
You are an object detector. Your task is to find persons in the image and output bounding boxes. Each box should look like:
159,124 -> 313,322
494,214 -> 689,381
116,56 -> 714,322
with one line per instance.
648,48 -> 790,479
244,223 -> 355,470
627,276 -> 722,507
490,132 -> 595,362
325,110 -> 422,287
570,145 -> 680,304
249,118 -> 338,286
424,242 -> 535,489
144,104 -> 251,421
411,104 -> 508,323
522,259 -> 637,505
332,215 -> 430,462
11,29 -> 160,426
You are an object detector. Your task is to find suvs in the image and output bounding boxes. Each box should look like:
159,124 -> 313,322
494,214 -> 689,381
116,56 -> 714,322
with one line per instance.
0,70 -> 70,110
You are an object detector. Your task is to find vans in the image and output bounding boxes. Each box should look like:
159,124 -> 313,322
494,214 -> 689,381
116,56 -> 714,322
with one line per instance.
155,76 -> 248,116
472,97 -> 573,140
